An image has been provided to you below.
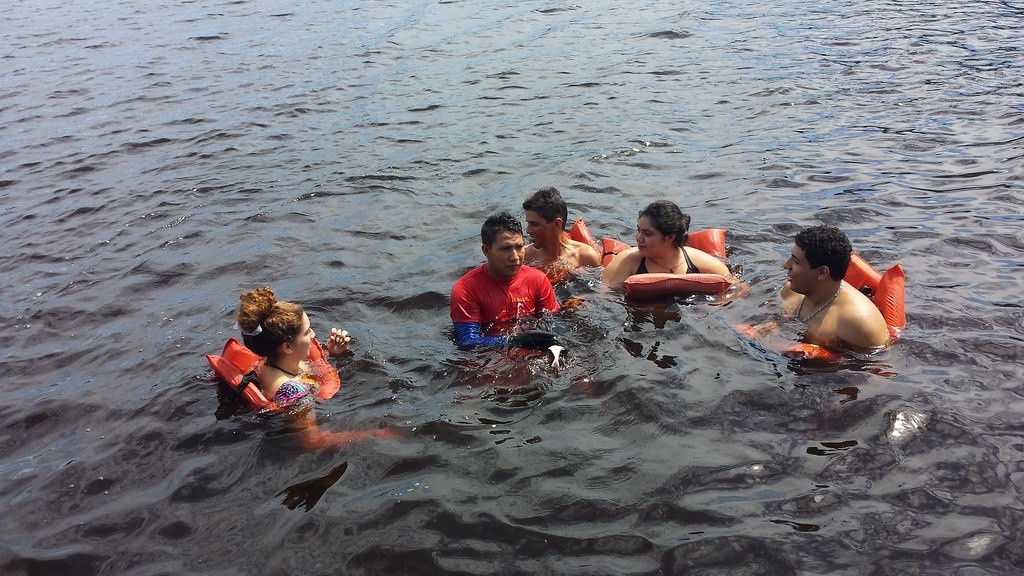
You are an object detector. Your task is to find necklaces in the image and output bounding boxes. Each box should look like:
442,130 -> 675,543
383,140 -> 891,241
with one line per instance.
795,278 -> 843,322
264,362 -> 304,376
647,257 -> 686,273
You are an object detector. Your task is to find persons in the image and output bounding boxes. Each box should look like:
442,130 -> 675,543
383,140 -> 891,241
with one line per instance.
594,199 -> 732,292
205,286 -> 404,451
777,224 -> 890,351
449,211 -> 561,351
522,187 -> 602,287
